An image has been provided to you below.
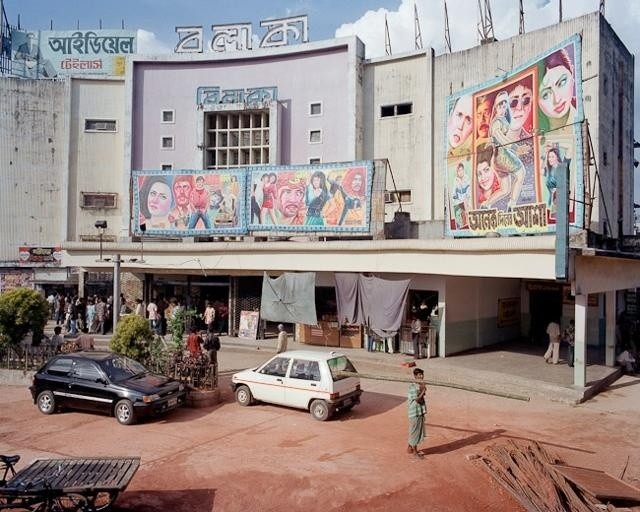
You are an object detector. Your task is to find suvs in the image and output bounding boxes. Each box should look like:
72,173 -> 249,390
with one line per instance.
229,350 -> 364,420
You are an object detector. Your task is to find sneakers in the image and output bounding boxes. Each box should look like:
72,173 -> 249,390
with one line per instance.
414,452 -> 425,458
407,447 -> 413,454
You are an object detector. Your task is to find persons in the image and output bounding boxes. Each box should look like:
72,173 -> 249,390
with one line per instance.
545,142 -> 573,211
474,75 -> 534,217
44,289 -> 228,337
447,96 -> 474,152
406,367 -> 428,457
30,328 -> 49,363
616,310 -> 640,376
136,166 -> 366,231
185,326 -> 205,388
536,46 -> 579,131
563,319 -> 574,368
451,162 -> 471,229
202,326 -> 221,388
411,312 -> 423,360
50,325 -> 65,352
13,31 -> 45,79
545,314 -> 563,365
275,323 -> 288,354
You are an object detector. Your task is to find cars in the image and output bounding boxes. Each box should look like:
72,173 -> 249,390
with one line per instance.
27,348 -> 186,424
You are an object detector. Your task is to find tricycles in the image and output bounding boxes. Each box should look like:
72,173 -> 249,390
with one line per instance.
0,453 -> 140,511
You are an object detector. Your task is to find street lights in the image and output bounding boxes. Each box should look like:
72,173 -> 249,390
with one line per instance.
94,219 -> 146,337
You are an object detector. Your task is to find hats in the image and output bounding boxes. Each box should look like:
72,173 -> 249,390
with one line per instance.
278,324 -> 284,331
